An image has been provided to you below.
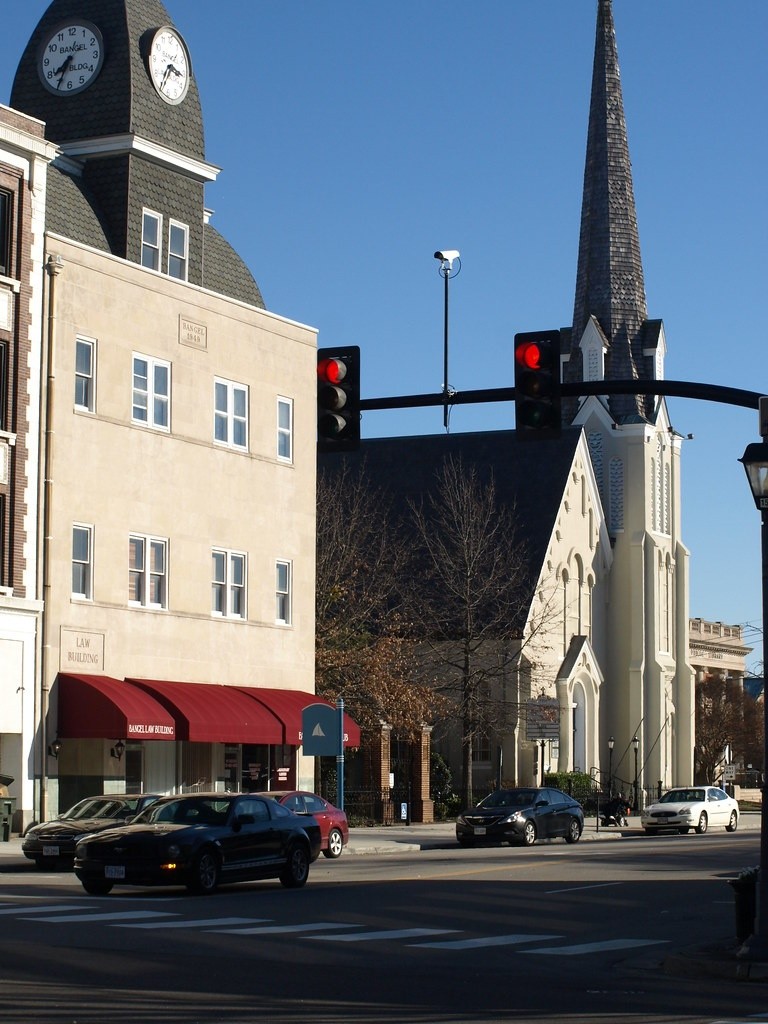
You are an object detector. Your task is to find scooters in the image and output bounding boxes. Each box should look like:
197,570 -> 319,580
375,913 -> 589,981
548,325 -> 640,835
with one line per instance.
598,806 -> 631,827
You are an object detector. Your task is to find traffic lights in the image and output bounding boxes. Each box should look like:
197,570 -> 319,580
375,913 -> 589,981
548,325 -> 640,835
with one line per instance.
317,347 -> 361,454
515,329 -> 562,438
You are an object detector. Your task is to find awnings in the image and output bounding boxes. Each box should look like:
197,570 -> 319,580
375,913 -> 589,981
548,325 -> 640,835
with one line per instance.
58,673 -> 175,741
225,685 -> 360,748
124,677 -> 283,745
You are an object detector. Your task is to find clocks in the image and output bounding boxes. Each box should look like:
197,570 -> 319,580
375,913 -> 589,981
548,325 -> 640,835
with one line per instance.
146,26 -> 193,105
37,19 -> 106,97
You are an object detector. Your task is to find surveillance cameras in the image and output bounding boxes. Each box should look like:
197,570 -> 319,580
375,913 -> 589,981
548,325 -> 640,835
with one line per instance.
433,250 -> 460,269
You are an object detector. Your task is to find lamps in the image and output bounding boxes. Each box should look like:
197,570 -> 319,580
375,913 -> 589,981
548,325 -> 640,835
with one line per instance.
48,739 -> 63,760
111,740 -> 125,762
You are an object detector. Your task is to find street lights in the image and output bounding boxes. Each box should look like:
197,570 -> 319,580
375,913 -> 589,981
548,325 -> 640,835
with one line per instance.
607,736 -> 615,802
738,442 -> 767,963
631,737 -> 640,810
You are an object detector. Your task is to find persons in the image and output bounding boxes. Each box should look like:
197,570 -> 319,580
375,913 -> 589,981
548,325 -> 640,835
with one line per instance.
699,791 -> 718,800
613,793 -> 631,827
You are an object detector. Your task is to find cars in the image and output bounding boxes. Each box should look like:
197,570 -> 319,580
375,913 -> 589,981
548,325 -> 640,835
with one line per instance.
21,792 -> 194,872
640,784 -> 740,836
455,788 -> 584,845
73,792 -> 323,896
217,790 -> 349,859
